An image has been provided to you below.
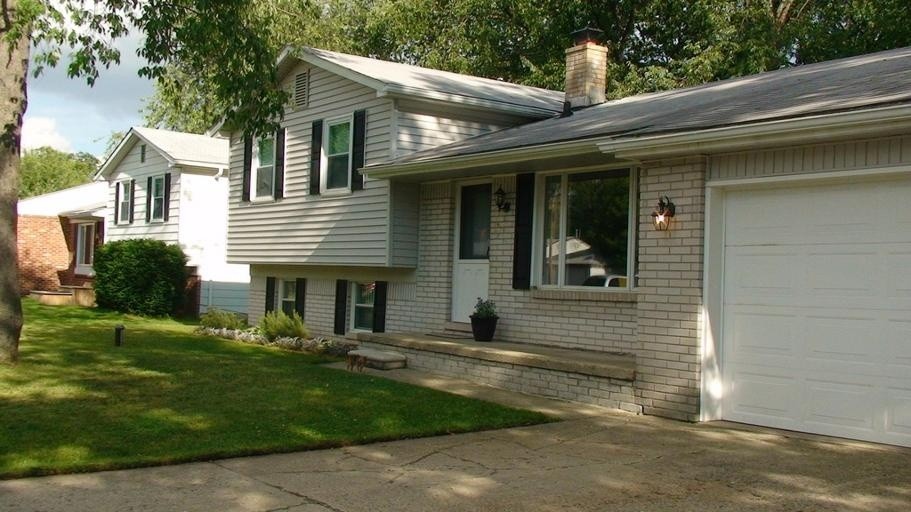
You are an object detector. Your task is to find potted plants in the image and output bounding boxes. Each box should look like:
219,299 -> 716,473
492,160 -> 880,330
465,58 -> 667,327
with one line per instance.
468,297 -> 499,342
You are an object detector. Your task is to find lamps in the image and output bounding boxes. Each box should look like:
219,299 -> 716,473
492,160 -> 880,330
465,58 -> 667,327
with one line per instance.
652,194 -> 675,231
